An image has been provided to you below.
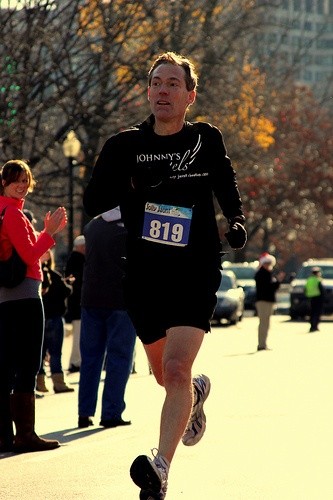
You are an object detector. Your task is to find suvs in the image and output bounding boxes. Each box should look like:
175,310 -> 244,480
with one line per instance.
223,260 -> 261,315
290,257 -> 333,320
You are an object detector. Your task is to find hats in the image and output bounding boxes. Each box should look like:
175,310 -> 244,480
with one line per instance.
312,267 -> 322,273
259,252 -> 276,266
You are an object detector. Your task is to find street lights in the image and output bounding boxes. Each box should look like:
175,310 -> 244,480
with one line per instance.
62,130 -> 83,252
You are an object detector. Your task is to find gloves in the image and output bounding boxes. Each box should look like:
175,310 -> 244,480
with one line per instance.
224,220 -> 248,249
132,159 -> 165,193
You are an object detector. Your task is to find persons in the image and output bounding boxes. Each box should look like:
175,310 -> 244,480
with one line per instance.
306,267 -> 326,332
253,254 -> 285,350
84,51 -> 248,500
36,249 -> 76,394
65,236 -> 137,429
0,159 -> 68,453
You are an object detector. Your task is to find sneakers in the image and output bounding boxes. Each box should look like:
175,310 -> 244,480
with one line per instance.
182,374 -> 211,446
130,448 -> 169,500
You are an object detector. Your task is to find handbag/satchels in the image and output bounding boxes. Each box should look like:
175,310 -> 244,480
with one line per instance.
0,208 -> 29,289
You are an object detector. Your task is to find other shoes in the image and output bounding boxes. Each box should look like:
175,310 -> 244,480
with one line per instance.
310,327 -> 320,332
67,363 -> 81,373
257,345 -> 268,351
99,414 -> 132,428
78,416 -> 94,428
34,390 -> 44,399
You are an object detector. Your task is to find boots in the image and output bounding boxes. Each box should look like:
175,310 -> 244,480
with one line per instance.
51,373 -> 75,394
36,374 -> 49,392
10,393 -> 60,453
0,392 -> 15,453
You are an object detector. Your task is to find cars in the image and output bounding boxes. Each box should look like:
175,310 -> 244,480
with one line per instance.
272,293 -> 290,314
212,270 -> 246,325
